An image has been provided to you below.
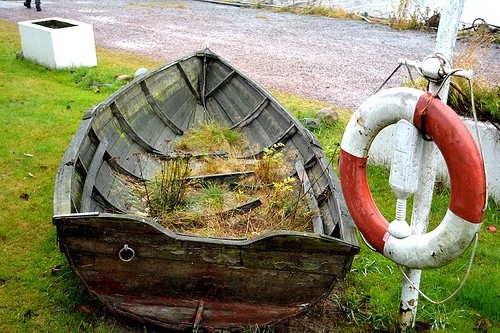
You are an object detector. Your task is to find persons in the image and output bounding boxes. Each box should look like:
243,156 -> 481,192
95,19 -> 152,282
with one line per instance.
24,0 -> 41,11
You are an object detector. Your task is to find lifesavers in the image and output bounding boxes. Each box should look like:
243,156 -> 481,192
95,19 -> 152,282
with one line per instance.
337,86 -> 486,271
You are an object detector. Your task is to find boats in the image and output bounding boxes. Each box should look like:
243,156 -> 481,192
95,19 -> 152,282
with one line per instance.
53,47 -> 360,332
